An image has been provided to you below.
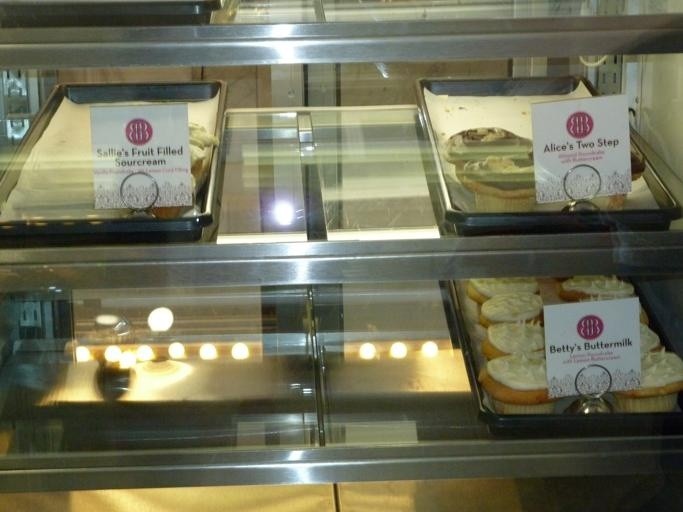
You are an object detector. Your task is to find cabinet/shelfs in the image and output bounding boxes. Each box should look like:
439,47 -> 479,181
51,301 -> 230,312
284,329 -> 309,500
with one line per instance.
2,1 -> 681,510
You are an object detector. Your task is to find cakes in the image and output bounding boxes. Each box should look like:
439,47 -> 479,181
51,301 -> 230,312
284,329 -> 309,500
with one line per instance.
443,127 -> 645,212
465,274 -> 683,414
